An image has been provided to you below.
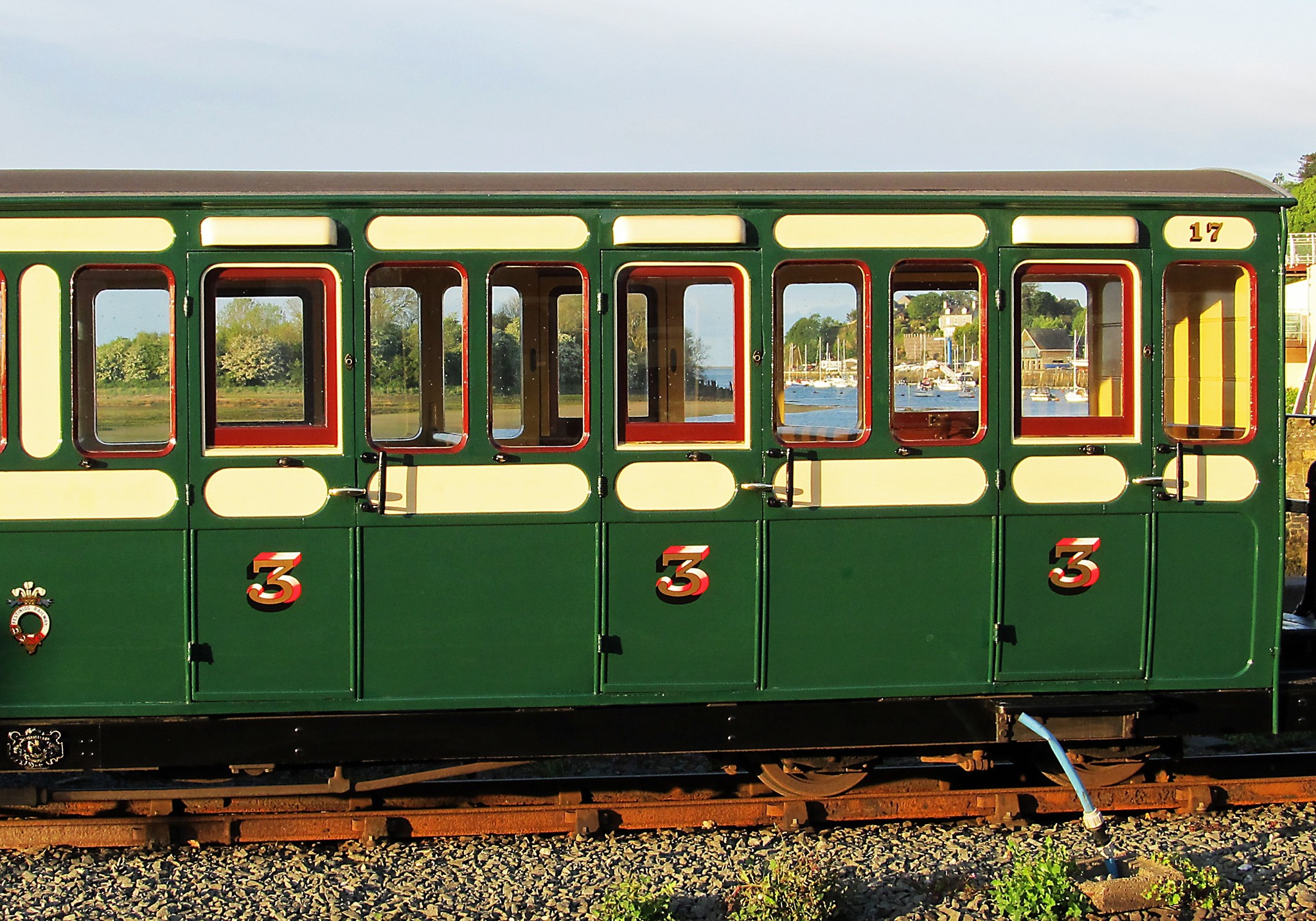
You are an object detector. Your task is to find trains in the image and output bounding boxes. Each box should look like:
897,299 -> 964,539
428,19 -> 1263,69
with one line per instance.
1,167 -> 1316,803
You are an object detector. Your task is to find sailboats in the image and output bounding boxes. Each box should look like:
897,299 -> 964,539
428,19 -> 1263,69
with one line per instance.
896,333 -> 976,398
1042,313 -> 1088,371
784,336 -> 858,388
1065,329 -> 1088,403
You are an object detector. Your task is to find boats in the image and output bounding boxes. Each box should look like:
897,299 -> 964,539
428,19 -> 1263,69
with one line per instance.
1028,386 -> 1060,402
